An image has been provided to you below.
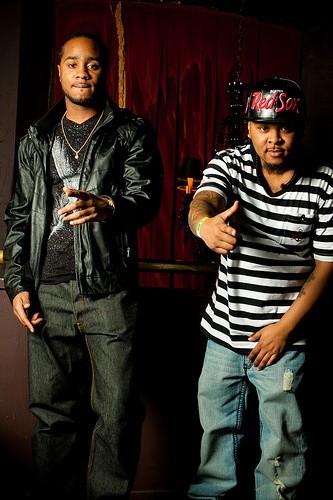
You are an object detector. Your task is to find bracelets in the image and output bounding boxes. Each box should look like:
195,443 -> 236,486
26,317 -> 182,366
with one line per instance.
196,216 -> 210,238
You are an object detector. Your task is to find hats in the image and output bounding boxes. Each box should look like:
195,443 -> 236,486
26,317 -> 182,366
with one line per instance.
244,77 -> 305,125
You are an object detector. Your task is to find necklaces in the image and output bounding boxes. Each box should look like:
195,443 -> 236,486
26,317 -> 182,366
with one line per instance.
61,109 -> 104,160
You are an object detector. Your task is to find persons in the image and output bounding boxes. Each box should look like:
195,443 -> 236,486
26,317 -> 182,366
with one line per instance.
188,77 -> 333,500
2,32 -> 163,500
